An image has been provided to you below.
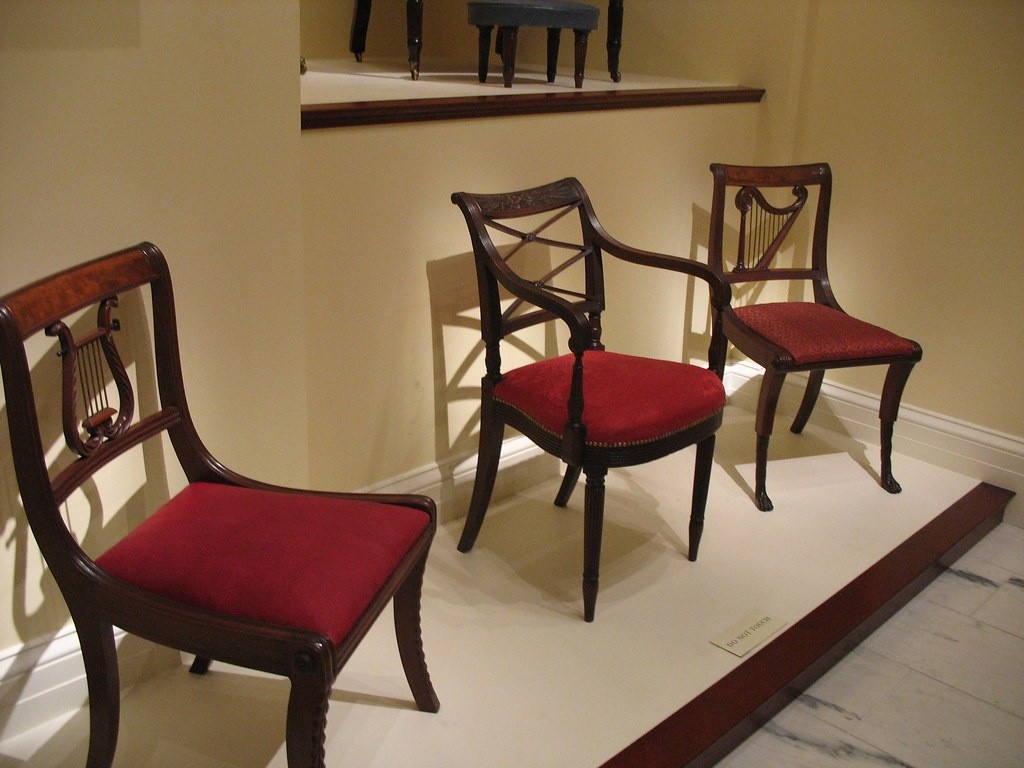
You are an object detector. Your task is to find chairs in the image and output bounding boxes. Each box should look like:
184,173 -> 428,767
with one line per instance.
709,162 -> 923,511
0,241 -> 440,768
451,177 -> 733,621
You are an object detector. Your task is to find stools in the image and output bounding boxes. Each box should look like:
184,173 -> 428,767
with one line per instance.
468,0 -> 600,89
